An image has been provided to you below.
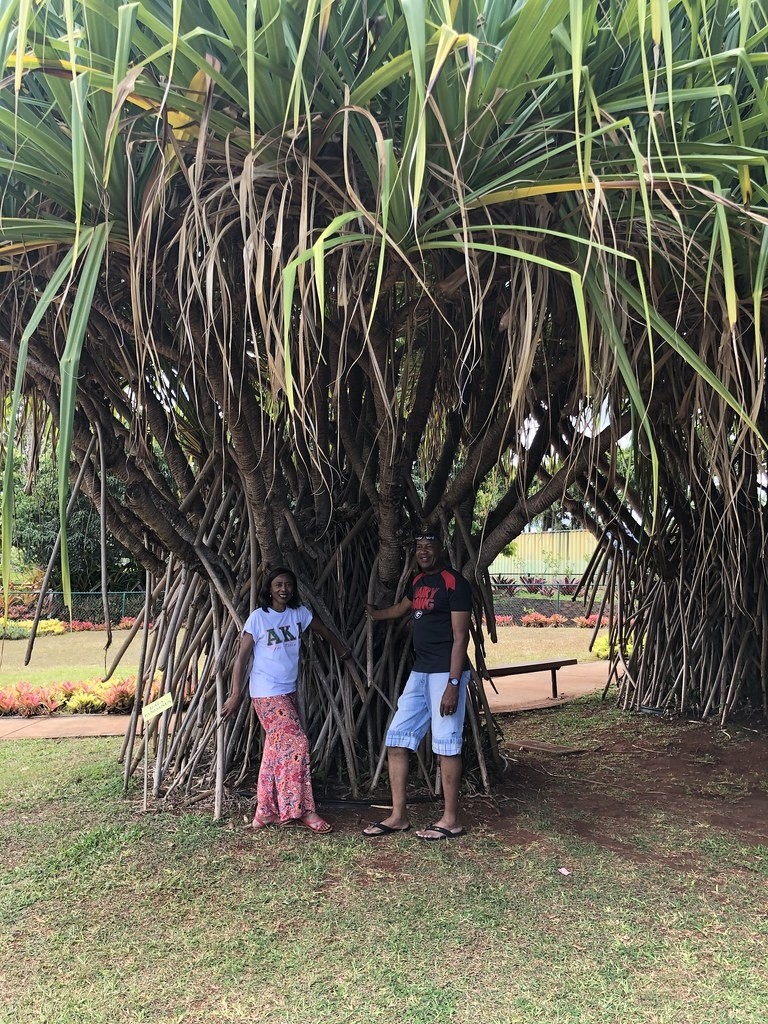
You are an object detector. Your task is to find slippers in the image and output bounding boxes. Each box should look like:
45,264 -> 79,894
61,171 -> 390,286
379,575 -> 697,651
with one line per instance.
416,824 -> 465,840
362,821 -> 410,837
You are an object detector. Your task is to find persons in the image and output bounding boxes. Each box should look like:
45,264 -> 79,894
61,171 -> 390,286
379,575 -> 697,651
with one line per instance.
220,567 -> 352,834
361,533 -> 473,840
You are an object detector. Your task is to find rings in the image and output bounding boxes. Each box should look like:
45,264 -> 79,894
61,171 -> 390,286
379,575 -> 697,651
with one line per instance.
449,711 -> 453,712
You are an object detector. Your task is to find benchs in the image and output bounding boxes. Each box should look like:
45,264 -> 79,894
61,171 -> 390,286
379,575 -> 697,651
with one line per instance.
473,658 -> 578,708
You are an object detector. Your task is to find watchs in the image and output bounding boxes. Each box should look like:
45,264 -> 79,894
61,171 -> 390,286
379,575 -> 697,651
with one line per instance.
448,678 -> 460,686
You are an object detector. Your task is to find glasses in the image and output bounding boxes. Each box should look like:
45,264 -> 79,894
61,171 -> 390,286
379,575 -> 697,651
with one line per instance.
414,533 -> 443,544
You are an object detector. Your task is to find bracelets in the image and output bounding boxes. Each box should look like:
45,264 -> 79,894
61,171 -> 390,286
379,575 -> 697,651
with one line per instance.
340,652 -> 346,658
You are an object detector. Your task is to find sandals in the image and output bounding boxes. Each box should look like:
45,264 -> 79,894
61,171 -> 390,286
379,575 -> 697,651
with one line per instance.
252,815 -> 295,830
296,812 -> 333,834
